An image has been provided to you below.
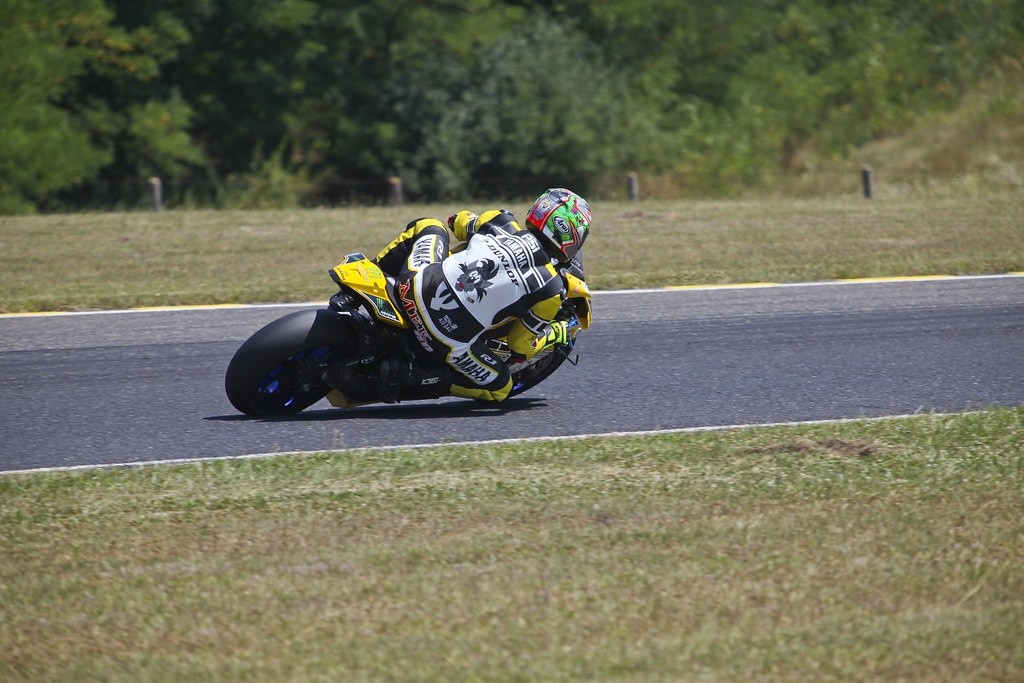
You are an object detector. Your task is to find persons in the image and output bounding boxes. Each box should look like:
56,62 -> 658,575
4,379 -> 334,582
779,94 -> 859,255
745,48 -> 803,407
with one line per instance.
329,186 -> 595,406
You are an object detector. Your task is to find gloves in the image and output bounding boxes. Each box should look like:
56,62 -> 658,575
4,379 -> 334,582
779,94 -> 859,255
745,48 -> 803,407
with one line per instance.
546,321 -> 571,349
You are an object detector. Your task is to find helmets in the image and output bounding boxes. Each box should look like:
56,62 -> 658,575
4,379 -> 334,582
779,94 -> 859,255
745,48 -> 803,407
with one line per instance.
525,188 -> 591,262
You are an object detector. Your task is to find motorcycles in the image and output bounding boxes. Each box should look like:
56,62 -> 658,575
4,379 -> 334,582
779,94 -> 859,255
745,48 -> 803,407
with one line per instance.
225,250 -> 594,420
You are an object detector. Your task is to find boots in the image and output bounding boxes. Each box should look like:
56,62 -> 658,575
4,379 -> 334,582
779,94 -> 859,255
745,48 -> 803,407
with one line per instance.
379,358 -> 451,399
